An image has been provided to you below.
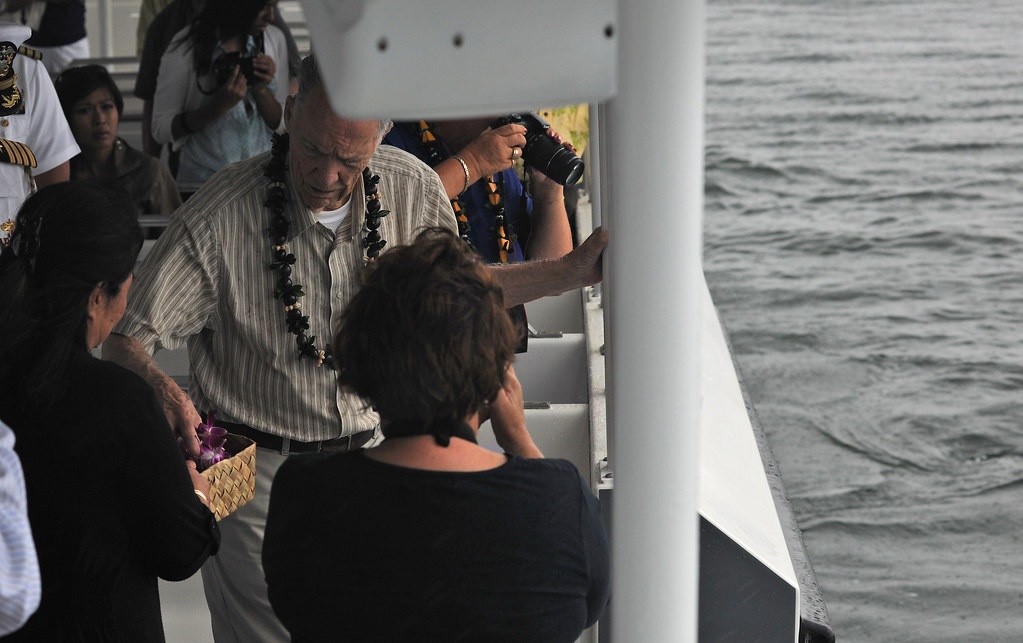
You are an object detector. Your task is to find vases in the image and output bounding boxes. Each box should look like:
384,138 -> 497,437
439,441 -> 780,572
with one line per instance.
177,430 -> 257,525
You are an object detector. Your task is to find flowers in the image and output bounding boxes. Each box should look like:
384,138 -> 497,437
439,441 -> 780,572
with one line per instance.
191,403 -> 234,470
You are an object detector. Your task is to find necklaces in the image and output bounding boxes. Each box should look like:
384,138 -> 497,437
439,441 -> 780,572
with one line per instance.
419,119 -> 512,264
267,132 -> 391,370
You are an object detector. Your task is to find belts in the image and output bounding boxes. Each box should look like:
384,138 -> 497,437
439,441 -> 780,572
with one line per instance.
198,410 -> 375,454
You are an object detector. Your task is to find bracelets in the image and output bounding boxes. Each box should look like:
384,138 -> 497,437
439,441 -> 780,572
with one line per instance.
193,489 -> 208,503
451,155 -> 470,197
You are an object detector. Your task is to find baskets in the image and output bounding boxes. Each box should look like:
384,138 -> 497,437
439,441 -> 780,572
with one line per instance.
176,426 -> 256,525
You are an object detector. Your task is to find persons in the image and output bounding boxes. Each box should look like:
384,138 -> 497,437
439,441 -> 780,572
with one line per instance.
55,63 -> 186,239
132,0 -> 303,157
103,50 -> 465,642
3,1 -> 90,74
151,1 -> 291,203
379,116 -> 577,334
258,222 -> 613,643
0,179 -> 223,642
0,43 -> 82,186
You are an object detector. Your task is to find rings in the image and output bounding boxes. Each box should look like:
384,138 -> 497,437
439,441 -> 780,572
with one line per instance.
512,148 -> 519,160
511,158 -> 516,169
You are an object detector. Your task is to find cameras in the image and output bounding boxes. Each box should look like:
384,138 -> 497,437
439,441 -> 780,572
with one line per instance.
490,112 -> 586,187
212,29 -> 264,85
